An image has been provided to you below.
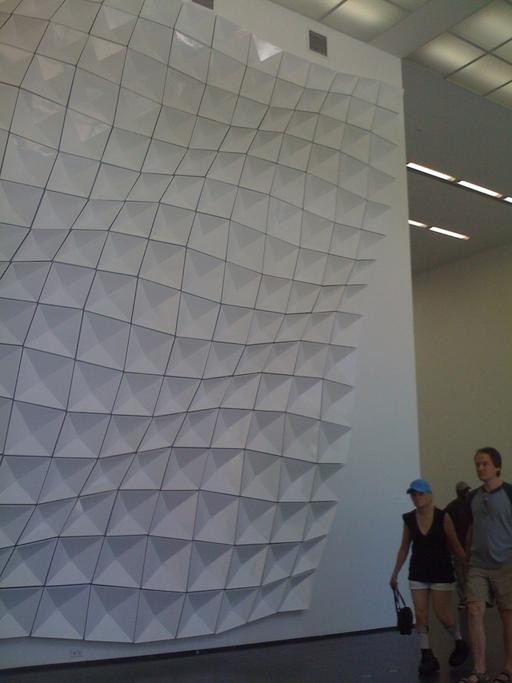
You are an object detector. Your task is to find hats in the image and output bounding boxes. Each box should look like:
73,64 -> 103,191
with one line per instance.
406,479 -> 432,493
456,481 -> 471,491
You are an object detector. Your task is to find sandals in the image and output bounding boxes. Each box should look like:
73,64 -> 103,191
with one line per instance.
458,670 -> 512,683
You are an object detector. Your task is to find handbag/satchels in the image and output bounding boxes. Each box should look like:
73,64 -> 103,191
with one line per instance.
398,607 -> 413,635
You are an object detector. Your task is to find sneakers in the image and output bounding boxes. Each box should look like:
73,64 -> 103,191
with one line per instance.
448,638 -> 469,666
418,648 -> 440,673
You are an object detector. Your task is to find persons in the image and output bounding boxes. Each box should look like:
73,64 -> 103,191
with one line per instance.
456,447 -> 512,683
443,481 -> 472,611
388,478 -> 470,674
